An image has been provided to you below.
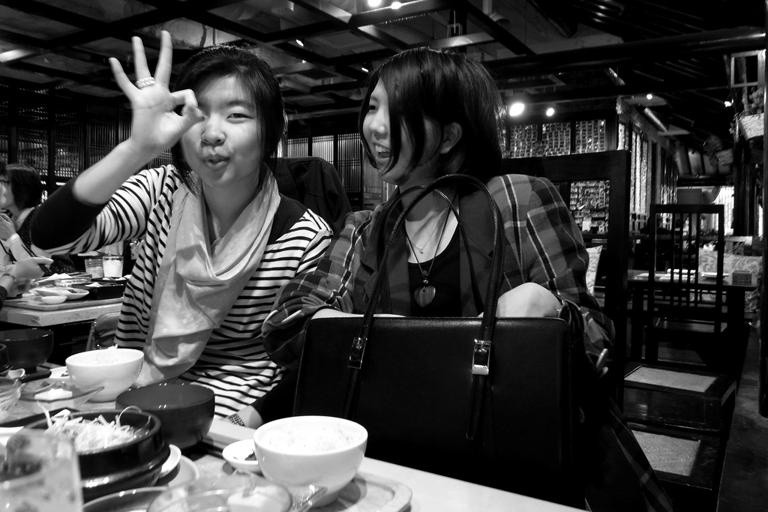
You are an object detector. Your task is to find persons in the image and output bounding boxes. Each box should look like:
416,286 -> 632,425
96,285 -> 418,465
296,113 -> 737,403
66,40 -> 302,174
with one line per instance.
254,46 -> 684,509
672,217 -> 688,241
0,159 -> 55,312
640,213 -> 673,261
1,162 -> 59,273
14,31 -> 336,431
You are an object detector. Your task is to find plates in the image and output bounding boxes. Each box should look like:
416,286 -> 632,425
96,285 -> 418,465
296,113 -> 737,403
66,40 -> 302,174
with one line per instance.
18,383 -> 105,410
223,440 -> 259,472
35,286 -> 90,301
42,295 -> 67,304
115,383 -> 215,451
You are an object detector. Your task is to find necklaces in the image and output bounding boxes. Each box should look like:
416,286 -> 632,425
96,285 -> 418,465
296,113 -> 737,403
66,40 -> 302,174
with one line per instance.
399,199 -> 454,309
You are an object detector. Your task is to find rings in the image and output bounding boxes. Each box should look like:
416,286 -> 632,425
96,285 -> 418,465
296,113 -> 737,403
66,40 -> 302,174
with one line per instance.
135,74 -> 156,88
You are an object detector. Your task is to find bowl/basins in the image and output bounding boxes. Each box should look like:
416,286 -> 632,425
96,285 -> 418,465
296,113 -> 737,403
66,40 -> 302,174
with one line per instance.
0,376 -> 21,420
80,486 -> 167,511
23,410 -> 170,507
0,328 -> 54,376
73,281 -> 126,300
148,474 -> 293,511
66,348 -> 145,402
55,278 -> 87,286
252,415 -> 368,506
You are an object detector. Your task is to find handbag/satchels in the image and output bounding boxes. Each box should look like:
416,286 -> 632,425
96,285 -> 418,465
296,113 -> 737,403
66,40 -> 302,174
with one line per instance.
294,173 -> 569,496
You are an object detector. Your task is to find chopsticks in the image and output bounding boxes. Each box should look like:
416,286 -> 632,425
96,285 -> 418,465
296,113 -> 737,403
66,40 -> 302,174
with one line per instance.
21,242 -> 53,276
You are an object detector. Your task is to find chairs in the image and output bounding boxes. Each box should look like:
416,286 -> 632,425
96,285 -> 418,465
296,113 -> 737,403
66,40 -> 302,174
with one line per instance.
646,204 -> 730,375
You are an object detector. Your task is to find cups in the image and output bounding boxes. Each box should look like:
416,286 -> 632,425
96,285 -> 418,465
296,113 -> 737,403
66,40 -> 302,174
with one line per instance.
85,259 -> 103,278
0,429 -> 82,510
103,256 -> 124,278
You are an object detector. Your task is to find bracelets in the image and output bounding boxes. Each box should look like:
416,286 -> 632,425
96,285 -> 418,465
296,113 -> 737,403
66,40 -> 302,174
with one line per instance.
2,272 -> 31,295
227,406 -> 247,425
